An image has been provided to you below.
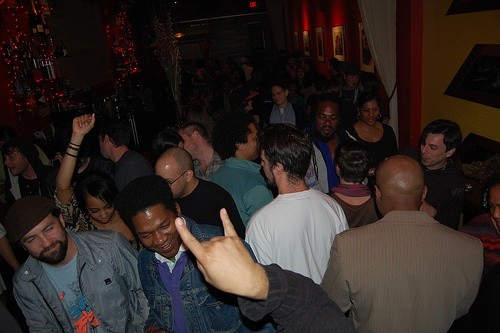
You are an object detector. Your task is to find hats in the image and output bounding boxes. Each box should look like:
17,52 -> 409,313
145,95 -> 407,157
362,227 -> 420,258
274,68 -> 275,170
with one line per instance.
6,194 -> 56,243
344,63 -> 365,77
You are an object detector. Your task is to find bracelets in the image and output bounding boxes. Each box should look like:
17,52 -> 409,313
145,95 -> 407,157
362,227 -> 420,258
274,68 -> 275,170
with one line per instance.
64,151 -> 78,158
69,141 -> 81,147
67,145 -> 80,151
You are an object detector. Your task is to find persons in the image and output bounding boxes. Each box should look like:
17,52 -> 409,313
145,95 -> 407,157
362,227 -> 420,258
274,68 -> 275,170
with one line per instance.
174,208 -> 358,333
0,54 -> 500,333
113,175 -> 280,333
320,156 -> 484,333
3,194 -> 150,333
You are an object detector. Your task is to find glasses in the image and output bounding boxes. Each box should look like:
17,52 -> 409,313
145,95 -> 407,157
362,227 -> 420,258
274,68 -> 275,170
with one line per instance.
167,171 -> 187,188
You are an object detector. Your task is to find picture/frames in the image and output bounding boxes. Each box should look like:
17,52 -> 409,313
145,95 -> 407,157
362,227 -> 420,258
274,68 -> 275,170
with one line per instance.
293,31 -> 299,49
359,22 -> 375,74
316,26 -> 324,62
332,25 -> 345,62
302,29 -> 311,57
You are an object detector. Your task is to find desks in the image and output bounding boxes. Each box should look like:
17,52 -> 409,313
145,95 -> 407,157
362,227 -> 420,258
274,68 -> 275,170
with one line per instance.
461,170 -> 490,225
